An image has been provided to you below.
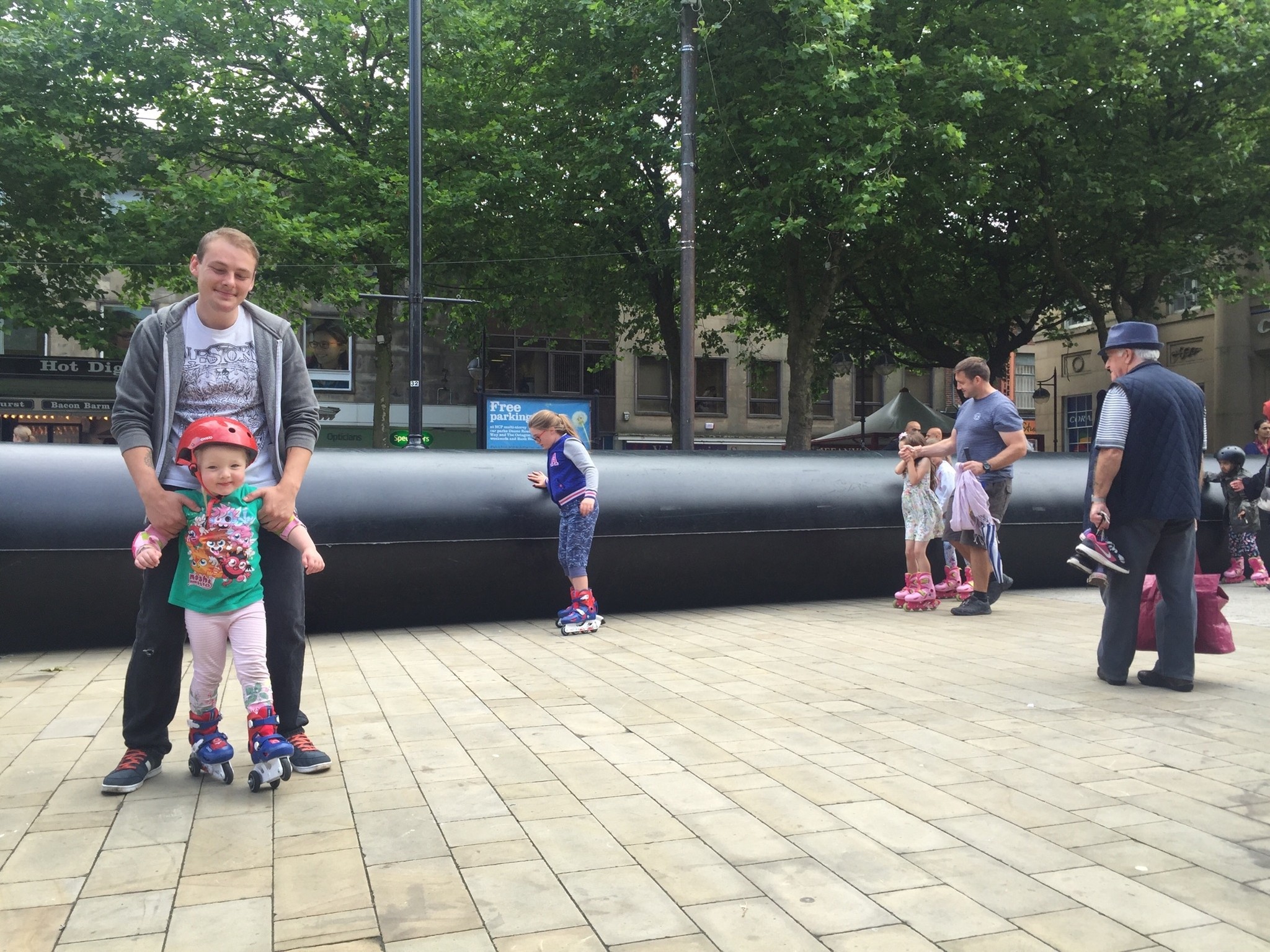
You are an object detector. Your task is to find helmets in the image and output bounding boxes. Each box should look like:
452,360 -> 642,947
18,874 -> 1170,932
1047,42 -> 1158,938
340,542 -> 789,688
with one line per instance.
1214,446 -> 1245,468
174,416 -> 261,466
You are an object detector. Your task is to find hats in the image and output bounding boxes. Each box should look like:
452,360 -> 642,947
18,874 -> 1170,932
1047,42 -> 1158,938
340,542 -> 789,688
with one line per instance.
1097,322 -> 1164,355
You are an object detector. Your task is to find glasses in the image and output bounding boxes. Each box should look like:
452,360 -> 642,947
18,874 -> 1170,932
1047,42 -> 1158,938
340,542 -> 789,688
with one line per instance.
533,430 -> 546,442
309,341 -> 340,349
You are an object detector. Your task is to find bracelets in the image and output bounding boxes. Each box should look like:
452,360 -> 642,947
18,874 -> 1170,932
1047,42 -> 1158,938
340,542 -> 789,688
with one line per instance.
1091,495 -> 1106,503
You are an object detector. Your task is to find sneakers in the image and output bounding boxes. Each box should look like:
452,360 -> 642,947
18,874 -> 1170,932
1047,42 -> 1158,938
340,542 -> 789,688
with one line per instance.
285,732 -> 331,773
951,596 -> 992,616
101,748 -> 161,794
987,573 -> 1013,604
1066,511 -> 1129,585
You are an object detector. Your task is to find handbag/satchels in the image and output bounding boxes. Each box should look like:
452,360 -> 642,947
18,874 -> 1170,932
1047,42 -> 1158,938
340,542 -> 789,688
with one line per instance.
1135,553 -> 1237,655
1258,459 -> 1270,512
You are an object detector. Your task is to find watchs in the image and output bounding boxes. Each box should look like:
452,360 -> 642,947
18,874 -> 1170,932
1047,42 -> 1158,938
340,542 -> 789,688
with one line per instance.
982,460 -> 991,473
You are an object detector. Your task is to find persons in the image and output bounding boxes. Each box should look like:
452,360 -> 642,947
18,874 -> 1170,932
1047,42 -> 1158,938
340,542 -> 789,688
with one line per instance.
99,226 -> 334,795
1202,444 -> 1269,588
13,425 -> 38,443
1065,321 -> 1208,692
132,418 -> 326,793
527,409 -> 606,635
1230,401 -> 1270,591
1244,418 -> 1270,456
106,310 -> 141,360
307,323 -> 350,371
889,356 -> 1025,616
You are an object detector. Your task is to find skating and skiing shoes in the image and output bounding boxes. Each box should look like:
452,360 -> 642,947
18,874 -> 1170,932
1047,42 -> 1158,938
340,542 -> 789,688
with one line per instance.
188,708 -> 235,784
555,585 -> 606,629
935,565 -> 962,599
903,572 -> 941,612
247,705 -> 294,791
892,571 -> 919,608
1224,557 -> 1246,582
1248,557 -> 1270,586
561,589 -> 601,635
956,566 -> 973,602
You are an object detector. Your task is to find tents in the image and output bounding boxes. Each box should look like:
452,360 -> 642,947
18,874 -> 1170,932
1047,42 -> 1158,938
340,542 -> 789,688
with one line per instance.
813,386 -> 957,450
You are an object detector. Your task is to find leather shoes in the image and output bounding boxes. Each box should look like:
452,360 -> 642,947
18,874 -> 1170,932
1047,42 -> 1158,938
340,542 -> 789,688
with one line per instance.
1097,666 -> 1126,686
1138,659 -> 1193,692
